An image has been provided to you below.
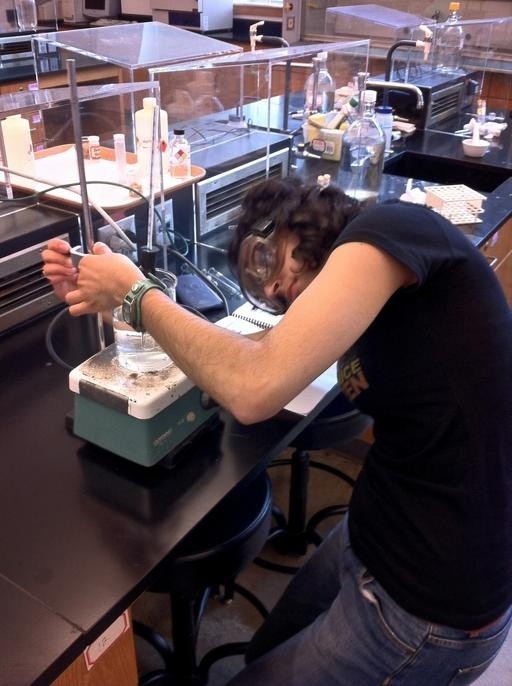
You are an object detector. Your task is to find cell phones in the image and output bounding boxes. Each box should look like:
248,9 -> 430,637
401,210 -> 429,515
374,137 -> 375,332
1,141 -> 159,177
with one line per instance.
173,274 -> 224,313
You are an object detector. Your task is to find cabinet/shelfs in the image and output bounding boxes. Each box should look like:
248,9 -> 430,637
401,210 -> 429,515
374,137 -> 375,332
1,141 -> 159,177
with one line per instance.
481,219 -> 511,310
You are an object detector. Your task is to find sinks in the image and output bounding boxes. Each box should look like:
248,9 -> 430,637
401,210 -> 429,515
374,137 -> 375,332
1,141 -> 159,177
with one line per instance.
380,149 -> 512,194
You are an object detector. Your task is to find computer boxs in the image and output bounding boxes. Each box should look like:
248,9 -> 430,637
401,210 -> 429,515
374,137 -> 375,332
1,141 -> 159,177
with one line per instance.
61,0 -> 88,24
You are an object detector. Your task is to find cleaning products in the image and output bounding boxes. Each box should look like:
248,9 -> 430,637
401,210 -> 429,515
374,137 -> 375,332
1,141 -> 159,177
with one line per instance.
133,97 -> 171,177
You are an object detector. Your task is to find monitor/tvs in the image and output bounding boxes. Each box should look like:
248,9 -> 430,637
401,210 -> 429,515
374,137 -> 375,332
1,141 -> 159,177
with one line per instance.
83,0 -> 120,21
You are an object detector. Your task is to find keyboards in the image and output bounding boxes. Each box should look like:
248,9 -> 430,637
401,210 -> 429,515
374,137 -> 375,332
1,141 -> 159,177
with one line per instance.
89,18 -> 138,27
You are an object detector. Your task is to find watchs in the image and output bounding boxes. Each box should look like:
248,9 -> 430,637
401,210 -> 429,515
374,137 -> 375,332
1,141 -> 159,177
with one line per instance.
122,279 -> 163,332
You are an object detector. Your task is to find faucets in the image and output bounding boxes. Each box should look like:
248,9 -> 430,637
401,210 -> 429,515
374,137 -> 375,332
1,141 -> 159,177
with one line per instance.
383,25 -> 434,106
365,80 -> 426,111
248,19 -> 292,131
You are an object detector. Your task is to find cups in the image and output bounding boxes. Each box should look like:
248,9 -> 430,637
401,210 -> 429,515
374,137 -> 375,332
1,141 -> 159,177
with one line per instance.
110,267 -> 178,374
462,138 -> 490,159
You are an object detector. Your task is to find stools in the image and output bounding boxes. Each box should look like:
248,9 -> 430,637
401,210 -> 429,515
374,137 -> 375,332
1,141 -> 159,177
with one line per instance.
252,394 -> 373,575
132,465 -> 275,685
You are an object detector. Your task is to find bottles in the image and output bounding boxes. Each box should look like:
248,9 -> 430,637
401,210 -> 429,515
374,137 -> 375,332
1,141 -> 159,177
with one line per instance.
80,93 -> 190,191
14,0 -> 38,31
296,56 -> 394,208
432,1 -> 466,73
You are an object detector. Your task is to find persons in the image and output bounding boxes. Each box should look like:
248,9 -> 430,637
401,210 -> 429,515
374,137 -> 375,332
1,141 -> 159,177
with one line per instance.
41,175 -> 512,686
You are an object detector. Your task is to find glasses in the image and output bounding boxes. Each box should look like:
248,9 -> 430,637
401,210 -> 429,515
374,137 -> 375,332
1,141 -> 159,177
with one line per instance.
238,203 -> 290,314
